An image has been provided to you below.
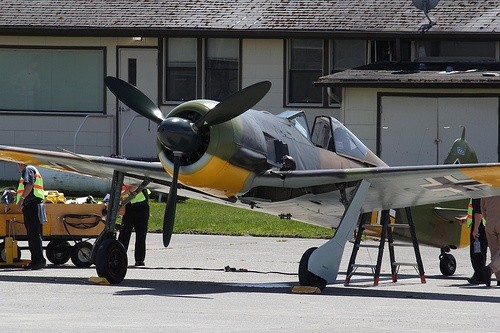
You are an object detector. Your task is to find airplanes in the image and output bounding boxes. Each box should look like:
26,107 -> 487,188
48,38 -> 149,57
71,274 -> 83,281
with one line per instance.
0,74 -> 500,293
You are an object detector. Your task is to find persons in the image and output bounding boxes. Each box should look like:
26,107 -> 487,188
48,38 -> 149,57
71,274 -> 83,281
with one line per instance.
117,183 -> 150,268
453,197 -> 489,285
13,162 -> 46,270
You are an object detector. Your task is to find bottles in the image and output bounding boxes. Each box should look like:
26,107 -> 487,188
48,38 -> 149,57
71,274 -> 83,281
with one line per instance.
473,234 -> 481,254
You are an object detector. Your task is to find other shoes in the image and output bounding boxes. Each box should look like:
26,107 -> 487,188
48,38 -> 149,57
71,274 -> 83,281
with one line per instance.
135,261 -> 145,266
20,260 -> 47,270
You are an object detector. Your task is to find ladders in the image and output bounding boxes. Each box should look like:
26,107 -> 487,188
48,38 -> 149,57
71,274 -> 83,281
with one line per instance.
344,207 -> 427,287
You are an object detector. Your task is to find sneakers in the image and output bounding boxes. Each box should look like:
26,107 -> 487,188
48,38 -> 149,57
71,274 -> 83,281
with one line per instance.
483,266 -> 492,286
